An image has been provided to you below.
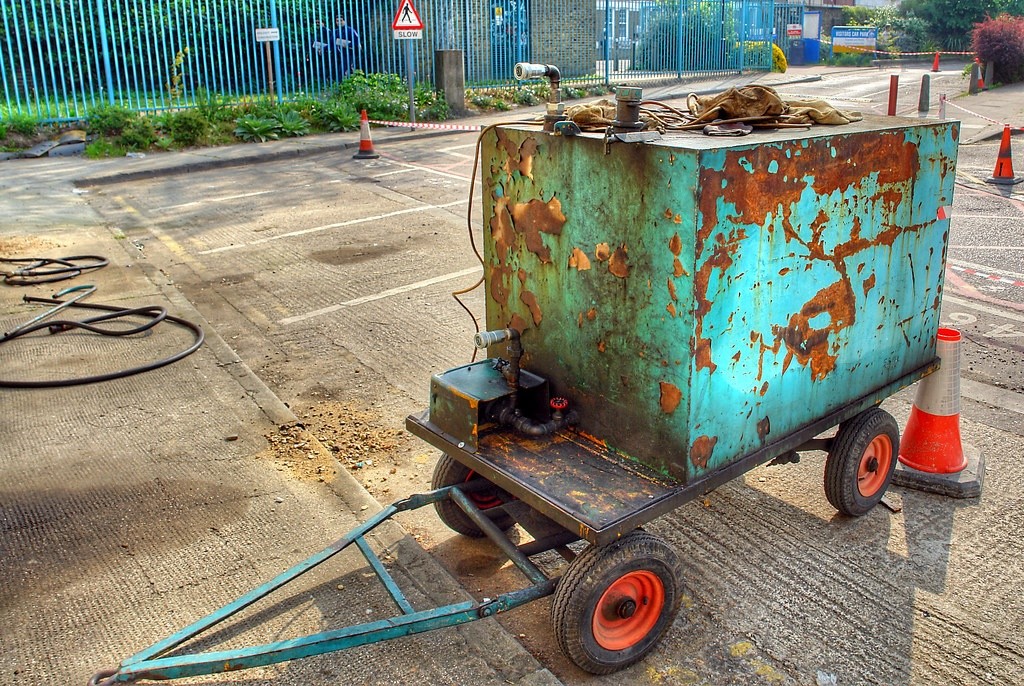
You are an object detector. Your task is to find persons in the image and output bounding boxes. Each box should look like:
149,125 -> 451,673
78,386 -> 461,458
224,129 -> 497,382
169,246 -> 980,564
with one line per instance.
331,15 -> 362,84
881,24 -> 892,58
306,17 -> 334,85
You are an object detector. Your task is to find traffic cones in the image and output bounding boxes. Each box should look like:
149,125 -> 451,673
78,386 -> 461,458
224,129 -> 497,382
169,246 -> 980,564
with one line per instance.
930,50 -> 941,71
975,57 -> 988,91
353,109 -> 380,159
984,123 -> 1024,185
890,327 -> 986,498
